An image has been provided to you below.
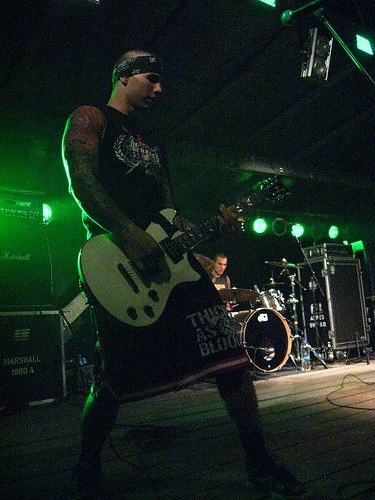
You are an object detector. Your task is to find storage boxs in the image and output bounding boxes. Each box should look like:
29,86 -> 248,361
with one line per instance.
0,310 -> 68,413
290,258 -> 370,364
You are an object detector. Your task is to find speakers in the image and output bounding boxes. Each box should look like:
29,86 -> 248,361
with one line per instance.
0,213 -> 54,309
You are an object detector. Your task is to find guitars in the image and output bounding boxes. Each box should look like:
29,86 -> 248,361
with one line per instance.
76,163 -> 315,334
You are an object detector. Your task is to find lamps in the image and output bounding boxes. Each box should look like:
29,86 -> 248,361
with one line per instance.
300,28 -> 334,81
248,216 -> 340,240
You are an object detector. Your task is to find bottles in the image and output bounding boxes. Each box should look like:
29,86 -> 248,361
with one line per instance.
328,342 -> 335,364
300,338 -> 311,372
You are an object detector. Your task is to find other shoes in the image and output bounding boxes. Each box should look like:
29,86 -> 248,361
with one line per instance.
62,457 -> 101,491
246,453 -> 308,495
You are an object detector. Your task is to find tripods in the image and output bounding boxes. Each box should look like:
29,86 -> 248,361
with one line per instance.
281,267 -> 328,372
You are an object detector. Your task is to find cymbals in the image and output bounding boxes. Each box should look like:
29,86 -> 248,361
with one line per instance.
261,282 -> 287,288
216,288 -> 259,302
264,261 -> 304,269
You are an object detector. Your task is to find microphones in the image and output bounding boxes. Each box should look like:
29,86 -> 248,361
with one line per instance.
280,0 -> 323,27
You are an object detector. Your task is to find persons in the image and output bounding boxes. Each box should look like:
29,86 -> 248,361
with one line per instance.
60,50 -> 308,500
207,252 -> 233,311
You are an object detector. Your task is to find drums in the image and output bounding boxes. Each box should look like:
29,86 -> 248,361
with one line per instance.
250,288 -> 287,316
227,308 -> 292,373
